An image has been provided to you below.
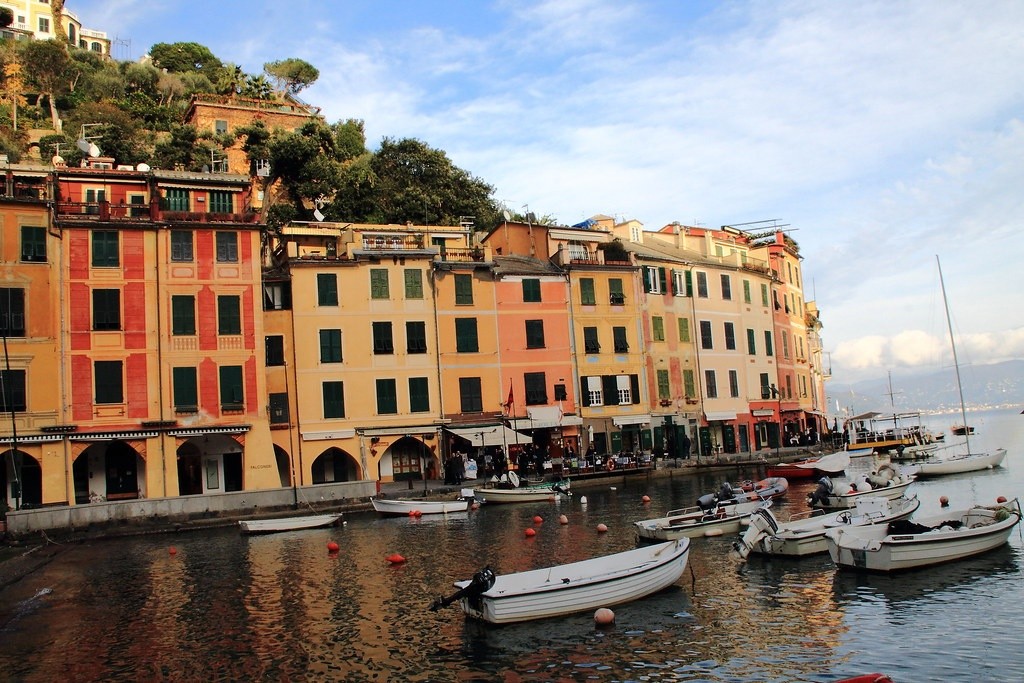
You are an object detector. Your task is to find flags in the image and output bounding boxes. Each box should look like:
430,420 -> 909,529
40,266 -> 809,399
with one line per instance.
499,378 -> 514,414
558,399 -> 565,424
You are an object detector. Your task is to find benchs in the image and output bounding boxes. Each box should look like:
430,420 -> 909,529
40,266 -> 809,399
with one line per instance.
563,453 -> 651,475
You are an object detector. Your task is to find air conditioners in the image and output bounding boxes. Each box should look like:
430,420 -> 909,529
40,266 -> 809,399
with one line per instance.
118,165 -> 132,171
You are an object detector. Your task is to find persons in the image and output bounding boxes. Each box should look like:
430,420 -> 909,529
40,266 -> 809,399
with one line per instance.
848,483 -> 858,494
585,441 -> 596,465
565,442 -> 574,458
471,245 -> 481,262
682,435 -> 691,459
444,439 -> 550,485
787,426 -> 816,447
769,383 -> 779,399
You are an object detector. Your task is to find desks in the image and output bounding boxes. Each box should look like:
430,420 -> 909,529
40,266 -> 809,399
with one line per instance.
543,463 -> 552,474
611,456 -> 619,470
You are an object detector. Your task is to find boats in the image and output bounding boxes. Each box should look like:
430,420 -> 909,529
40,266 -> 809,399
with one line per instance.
824,498 -> 1021,569
765,451 -> 850,480
428,537 -> 691,624
473,478 -> 573,504
633,476 -> 789,540
808,476 -> 916,508
733,496 -> 920,560
370,497 -> 469,514
238,513 -> 343,533
843,255 -> 1007,477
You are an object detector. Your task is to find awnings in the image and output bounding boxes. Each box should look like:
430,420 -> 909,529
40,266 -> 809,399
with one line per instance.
508,415 -> 583,429
443,424 -> 532,446
612,415 -> 651,427
704,410 -> 737,421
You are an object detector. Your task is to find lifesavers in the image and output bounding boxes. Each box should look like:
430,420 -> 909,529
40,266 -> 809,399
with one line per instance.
607,460 -> 614,470
